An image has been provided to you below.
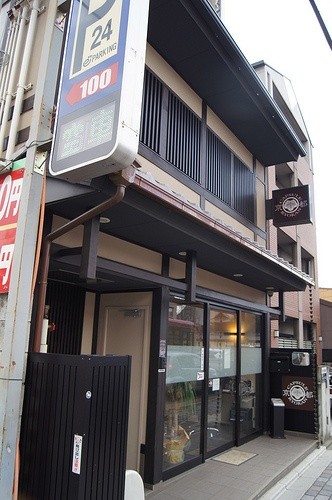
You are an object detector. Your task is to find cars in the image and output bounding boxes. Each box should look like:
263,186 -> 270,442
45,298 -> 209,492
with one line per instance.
166,352 -> 217,401
